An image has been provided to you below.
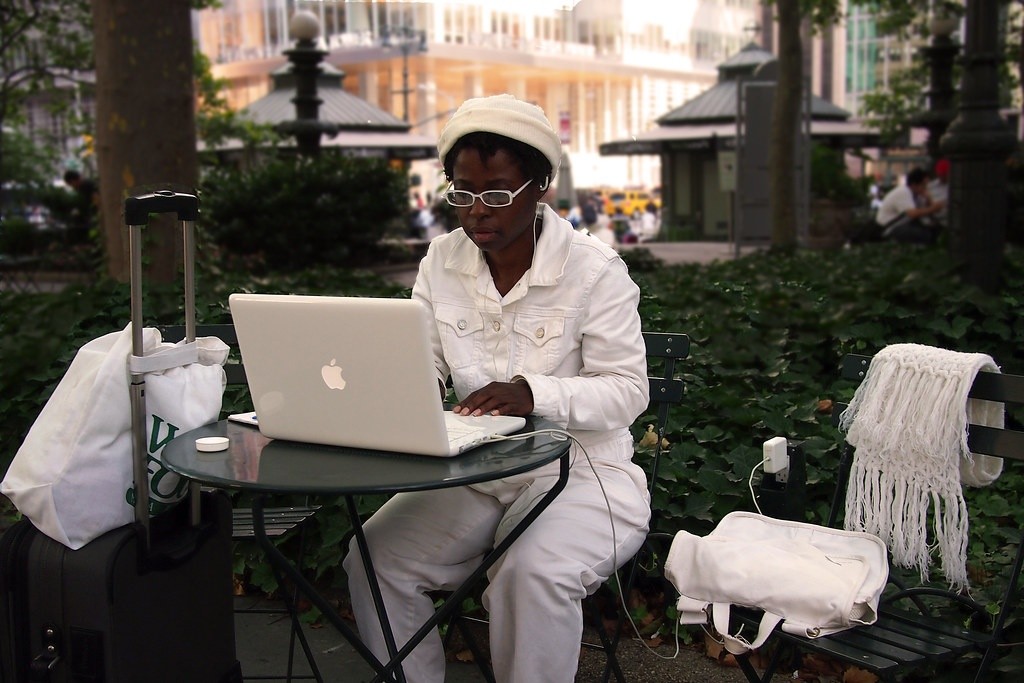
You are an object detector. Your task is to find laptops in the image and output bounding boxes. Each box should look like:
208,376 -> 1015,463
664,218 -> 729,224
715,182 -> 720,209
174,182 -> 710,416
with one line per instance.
228,293 -> 527,458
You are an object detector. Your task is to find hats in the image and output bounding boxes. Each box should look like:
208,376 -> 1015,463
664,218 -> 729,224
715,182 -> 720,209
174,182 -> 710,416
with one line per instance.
437,93 -> 562,182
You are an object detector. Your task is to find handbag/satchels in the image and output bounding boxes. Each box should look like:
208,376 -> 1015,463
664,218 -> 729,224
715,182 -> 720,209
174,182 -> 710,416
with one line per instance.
1,322 -> 230,550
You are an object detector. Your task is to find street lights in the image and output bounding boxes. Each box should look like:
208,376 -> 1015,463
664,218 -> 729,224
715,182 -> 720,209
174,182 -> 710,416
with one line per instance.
908,7 -> 966,158
277,10 -> 339,164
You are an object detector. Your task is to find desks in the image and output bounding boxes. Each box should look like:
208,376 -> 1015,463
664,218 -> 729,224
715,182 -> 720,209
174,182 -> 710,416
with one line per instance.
162,411 -> 571,683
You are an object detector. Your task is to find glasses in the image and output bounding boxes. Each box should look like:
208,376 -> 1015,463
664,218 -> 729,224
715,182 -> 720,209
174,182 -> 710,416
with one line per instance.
441,177 -> 534,207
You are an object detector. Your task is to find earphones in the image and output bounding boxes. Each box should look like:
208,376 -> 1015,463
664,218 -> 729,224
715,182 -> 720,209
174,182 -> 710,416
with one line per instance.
540,175 -> 550,192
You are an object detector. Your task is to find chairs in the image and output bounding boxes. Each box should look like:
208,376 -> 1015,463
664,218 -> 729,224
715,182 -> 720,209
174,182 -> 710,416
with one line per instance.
713,354 -> 1023,683
439,331 -> 694,683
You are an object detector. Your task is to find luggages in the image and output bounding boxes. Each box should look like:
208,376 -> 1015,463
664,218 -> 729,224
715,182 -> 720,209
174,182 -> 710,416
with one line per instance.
0,189 -> 244,683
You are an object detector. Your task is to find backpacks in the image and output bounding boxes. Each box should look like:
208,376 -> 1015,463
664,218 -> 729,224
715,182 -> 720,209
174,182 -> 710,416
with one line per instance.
664,511 -> 889,655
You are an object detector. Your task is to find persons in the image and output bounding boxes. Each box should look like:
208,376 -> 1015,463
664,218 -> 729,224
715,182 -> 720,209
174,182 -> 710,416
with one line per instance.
568,197 -> 658,246
869,165 -> 949,242
348,94 -> 652,683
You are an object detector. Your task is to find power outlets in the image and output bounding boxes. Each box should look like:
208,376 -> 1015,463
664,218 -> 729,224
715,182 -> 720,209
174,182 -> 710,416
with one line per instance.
776,452 -> 790,483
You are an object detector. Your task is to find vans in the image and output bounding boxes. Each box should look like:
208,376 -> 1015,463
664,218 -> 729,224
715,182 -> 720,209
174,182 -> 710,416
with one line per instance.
605,191 -> 662,219
